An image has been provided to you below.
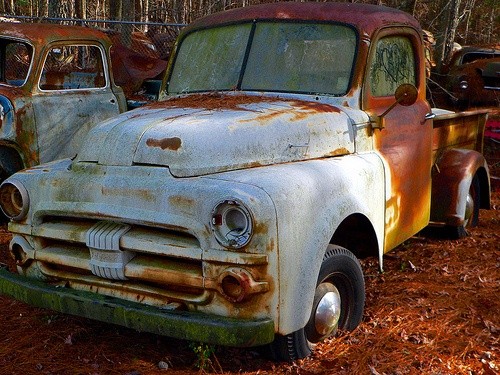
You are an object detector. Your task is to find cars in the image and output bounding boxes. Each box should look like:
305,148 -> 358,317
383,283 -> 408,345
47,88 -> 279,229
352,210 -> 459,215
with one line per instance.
429,45 -> 500,107
1,18 -> 126,180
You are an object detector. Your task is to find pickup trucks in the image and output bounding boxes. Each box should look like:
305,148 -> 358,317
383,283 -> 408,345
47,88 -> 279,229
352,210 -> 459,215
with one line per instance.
0,0 -> 493,365
